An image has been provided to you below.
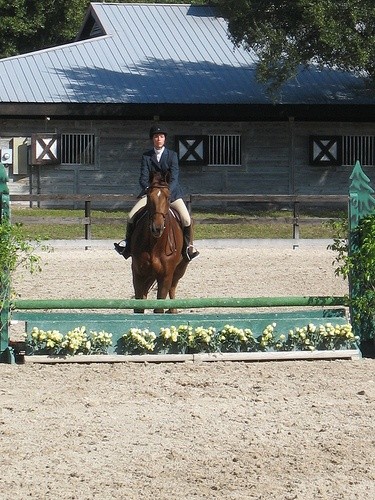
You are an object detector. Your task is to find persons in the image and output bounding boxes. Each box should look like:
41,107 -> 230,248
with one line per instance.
114,124 -> 200,262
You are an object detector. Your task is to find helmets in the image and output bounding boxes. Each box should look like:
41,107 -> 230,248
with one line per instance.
150,125 -> 167,135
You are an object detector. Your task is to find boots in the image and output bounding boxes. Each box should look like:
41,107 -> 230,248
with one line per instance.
114,221 -> 133,259
183,224 -> 199,259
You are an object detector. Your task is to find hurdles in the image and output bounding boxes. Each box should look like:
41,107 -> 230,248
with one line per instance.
10,295 -> 362,362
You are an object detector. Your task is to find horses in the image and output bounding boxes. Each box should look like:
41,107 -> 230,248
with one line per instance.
129,169 -> 193,313
